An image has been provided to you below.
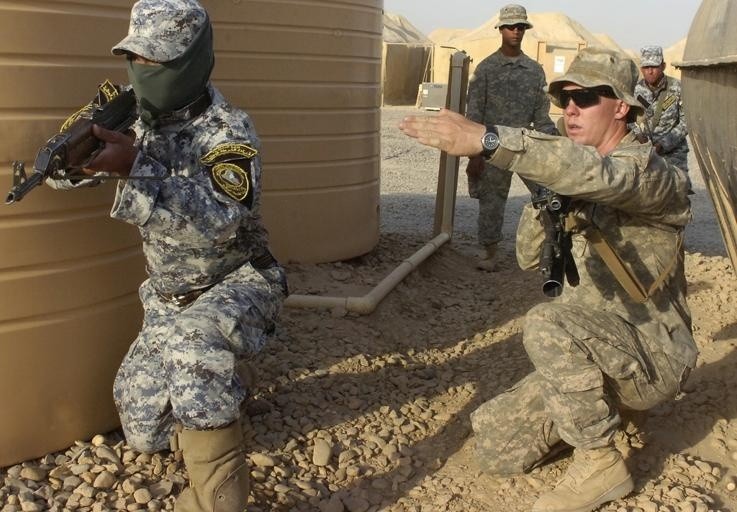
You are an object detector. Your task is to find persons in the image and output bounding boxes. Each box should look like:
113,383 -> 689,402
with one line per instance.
399,46 -> 698,512
632,44 -> 690,175
43,0 -> 287,512
465,5 -> 559,270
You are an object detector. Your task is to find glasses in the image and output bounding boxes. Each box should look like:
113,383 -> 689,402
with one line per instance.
559,87 -> 613,109
501,25 -> 524,32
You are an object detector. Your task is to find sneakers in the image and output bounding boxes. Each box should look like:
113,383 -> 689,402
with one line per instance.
531,446 -> 634,512
618,403 -> 647,437
473,245 -> 497,271
236,359 -> 257,413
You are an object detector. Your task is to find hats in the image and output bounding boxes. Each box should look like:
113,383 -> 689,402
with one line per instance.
638,45 -> 662,68
542,46 -> 647,117
111,0 -> 209,63
493,4 -> 533,30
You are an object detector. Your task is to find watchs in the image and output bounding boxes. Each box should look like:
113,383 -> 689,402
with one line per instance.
481,123 -> 500,157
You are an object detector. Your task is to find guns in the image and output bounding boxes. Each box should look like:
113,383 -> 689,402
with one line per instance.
5,83 -> 139,206
532,187 -> 580,299
